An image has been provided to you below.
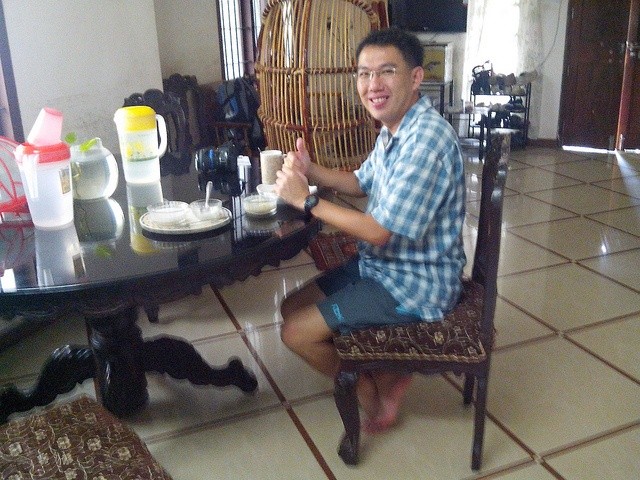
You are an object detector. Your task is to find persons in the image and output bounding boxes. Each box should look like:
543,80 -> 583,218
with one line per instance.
272,29 -> 467,436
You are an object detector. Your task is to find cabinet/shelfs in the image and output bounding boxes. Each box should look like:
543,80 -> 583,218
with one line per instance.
468,82 -> 531,149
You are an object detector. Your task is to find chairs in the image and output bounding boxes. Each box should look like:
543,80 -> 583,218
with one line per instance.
332,134 -> 511,470
122,90 -> 187,150
212,122 -> 252,153
0,392 -> 173,480
163,73 -> 206,147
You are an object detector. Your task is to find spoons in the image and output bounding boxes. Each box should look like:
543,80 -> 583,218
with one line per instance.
205,181 -> 213,208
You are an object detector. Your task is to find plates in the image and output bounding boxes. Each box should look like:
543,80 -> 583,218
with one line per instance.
139,207 -> 232,234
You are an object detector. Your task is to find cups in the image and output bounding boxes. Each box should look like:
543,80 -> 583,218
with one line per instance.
26,107 -> 63,149
260,150 -> 287,184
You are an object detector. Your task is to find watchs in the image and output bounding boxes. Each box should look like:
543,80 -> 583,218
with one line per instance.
305,195 -> 322,218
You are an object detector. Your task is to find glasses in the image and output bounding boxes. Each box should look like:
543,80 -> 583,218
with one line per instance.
353,65 -> 396,80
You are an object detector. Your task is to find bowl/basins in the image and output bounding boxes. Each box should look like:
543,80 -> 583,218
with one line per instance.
243,220 -> 280,238
147,201 -> 189,224
245,196 -> 277,212
190,199 -> 222,220
152,241 -> 191,251
256,184 -> 277,199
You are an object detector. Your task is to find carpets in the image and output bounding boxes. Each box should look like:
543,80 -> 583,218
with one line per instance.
310,229 -> 360,273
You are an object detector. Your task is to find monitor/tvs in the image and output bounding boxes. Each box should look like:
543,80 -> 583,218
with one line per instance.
389,1 -> 471,34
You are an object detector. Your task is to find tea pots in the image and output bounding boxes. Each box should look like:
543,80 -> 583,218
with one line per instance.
35,228 -> 86,287
126,183 -> 165,255
73,137 -> 118,200
73,199 -> 125,257
114,106 -> 166,183
14,143 -> 74,228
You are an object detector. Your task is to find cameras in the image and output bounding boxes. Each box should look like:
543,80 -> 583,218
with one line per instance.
195,140 -> 239,175
